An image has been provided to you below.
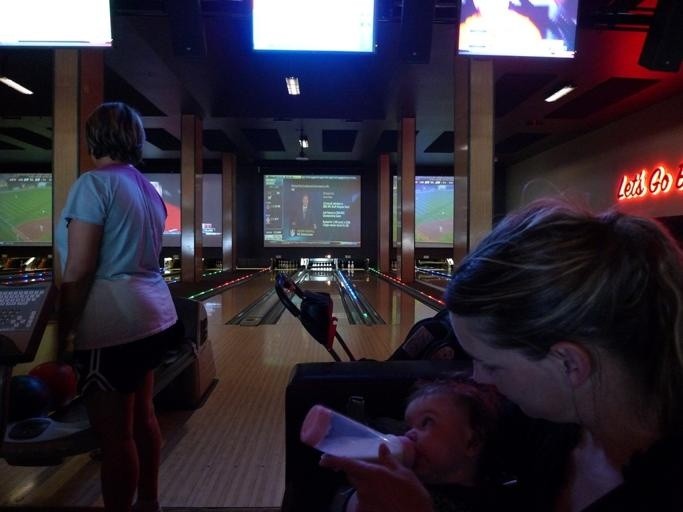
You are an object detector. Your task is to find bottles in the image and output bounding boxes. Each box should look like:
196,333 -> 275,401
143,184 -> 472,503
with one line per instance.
298,406 -> 415,471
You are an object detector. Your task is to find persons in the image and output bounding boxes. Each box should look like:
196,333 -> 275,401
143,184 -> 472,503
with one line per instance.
316,189 -> 682,511
291,192 -> 316,231
326,370 -> 504,510
45,97 -> 182,510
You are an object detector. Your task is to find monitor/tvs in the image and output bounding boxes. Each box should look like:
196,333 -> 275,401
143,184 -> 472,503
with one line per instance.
452,0 -> 581,64
0,2 -> 116,55
231,0 -> 401,63
